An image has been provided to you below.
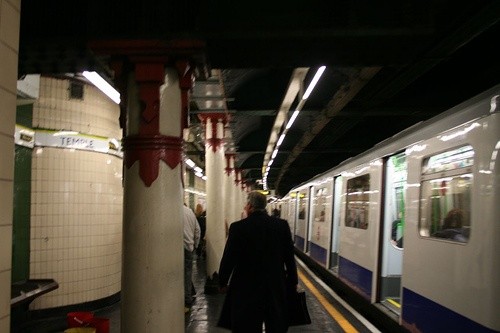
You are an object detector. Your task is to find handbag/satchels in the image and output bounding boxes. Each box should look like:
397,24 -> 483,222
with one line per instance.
288,292 -> 311,326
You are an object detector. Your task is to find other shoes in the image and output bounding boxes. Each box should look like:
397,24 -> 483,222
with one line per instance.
185,307 -> 192,312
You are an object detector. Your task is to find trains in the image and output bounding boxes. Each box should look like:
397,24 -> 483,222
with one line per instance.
277,84 -> 500,333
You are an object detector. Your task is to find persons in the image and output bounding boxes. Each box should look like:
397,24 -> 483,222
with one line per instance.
217,192 -> 299,333
183,202 -> 207,314
320,211 -> 325,221
431,207 -> 468,244
